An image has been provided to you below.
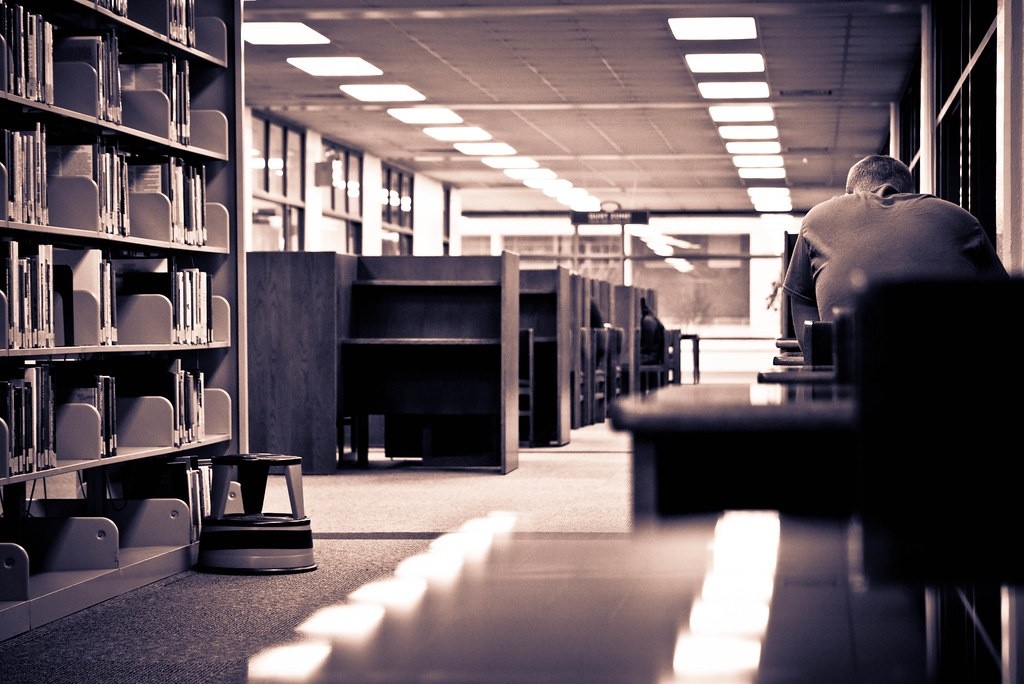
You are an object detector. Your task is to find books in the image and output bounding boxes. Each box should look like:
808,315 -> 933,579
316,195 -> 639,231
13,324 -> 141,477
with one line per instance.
47,131 -> 130,235
115,50 -> 192,146
54,244 -> 119,347
130,151 -> 209,247
0,235 -> 54,350
0,361 -> 59,478
0,120 -> 51,226
105,250 -> 213,346
49,26 -> 123,124
61,373 -> 118,461
1,2 -> 55,105
90,0 -> 197,48
115,357 -> 206,448
121,454 -> 215,545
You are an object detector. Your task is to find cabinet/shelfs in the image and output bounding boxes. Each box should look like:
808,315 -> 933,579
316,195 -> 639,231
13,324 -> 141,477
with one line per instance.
0,0 -> 249,641
336,251 -> 519,476
517,265 -> 658,445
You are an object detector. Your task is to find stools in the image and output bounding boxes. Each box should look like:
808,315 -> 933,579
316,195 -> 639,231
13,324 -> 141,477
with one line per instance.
199,453 -> 318,574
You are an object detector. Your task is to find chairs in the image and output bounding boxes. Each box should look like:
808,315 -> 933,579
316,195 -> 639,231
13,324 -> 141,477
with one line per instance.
833,307 -> 855,384
856,266 -> 1024,681
640,329 -> 680,395
803,319 -> 833,365
519,328 -> 536,441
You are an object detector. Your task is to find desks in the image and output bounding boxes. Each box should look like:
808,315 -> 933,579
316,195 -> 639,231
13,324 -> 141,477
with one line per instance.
609,383 -> 858,521
756,338 -> 834,402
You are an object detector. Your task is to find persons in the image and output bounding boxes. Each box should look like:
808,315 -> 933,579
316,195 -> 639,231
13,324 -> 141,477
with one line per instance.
780,153 -> 1011,371
590,297 -> 607,369
638,296 -> 667,367
